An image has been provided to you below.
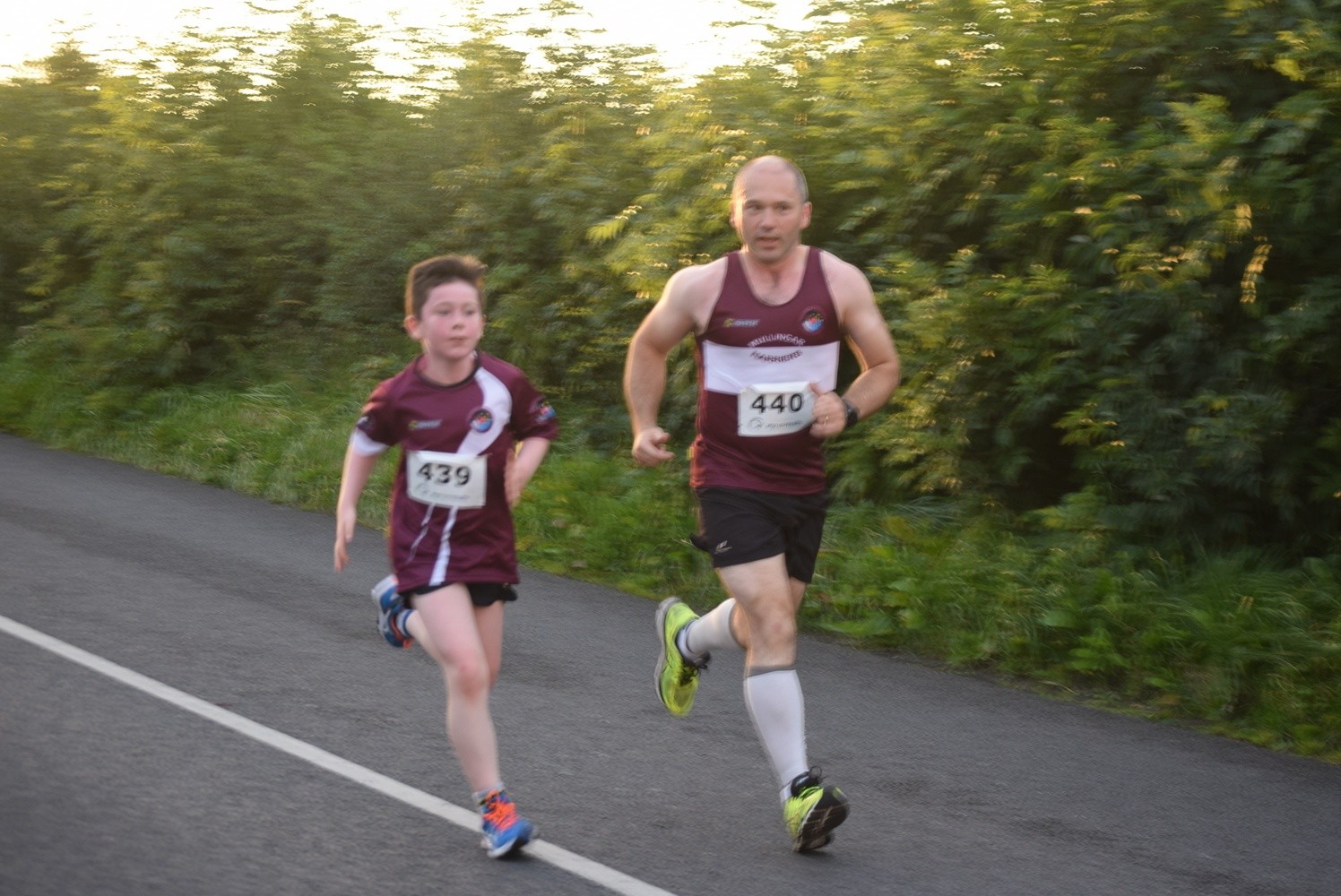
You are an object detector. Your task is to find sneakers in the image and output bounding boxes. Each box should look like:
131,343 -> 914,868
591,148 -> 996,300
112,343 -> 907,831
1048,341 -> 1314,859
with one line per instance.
655,597 -> 714,718
373,574 -> 416,649
783,768 -> 851,852
480,791 -> 538,860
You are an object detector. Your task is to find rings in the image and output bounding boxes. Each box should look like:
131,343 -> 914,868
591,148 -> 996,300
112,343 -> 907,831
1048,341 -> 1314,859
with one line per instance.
824,415 -> 829,424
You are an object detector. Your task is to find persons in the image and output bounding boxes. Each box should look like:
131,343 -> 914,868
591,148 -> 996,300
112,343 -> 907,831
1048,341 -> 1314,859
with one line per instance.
333,254 -> 559,859
622,156 -> 901,851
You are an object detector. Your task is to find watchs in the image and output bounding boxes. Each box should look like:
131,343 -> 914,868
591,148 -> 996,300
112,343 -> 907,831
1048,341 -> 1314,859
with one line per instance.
840,399 -> 860,430
